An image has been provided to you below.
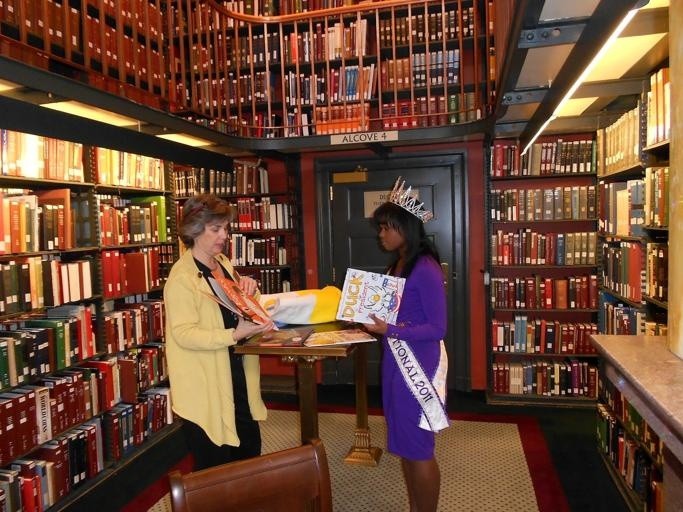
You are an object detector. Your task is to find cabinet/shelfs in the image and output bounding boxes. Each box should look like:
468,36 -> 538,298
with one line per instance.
482,38 -> 676,409
592,376 -> 683,512
1,0 -> 298,512
295,0 -> 492,129
